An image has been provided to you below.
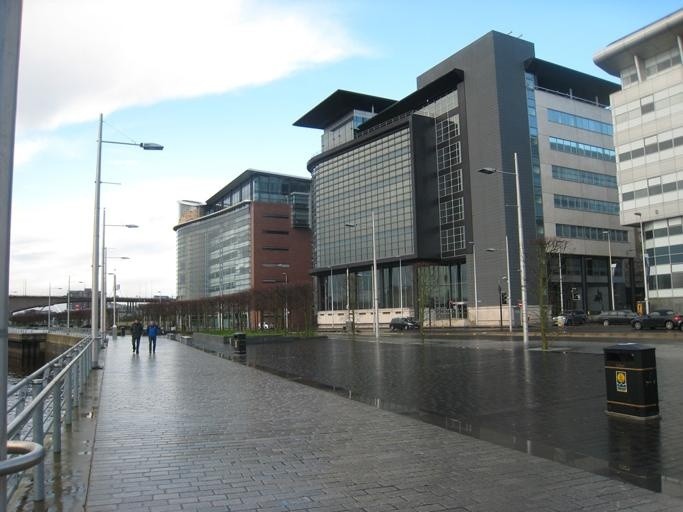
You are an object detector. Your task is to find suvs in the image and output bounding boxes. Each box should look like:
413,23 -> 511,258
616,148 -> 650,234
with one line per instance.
551,309 -> 586,327
389,316 -> 420,330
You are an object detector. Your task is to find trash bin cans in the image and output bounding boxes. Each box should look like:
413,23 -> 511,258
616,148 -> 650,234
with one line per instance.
120,327 -> 125,336
602,347 -> 659,418
233,334 -> 246,354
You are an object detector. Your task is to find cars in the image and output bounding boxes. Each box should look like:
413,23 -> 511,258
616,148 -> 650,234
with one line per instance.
591,307 -> 641,327
627,309 -> 683,329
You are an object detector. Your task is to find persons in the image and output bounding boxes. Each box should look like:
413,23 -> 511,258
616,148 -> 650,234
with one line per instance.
131,320 -> 143,354
147,320 -> 159,353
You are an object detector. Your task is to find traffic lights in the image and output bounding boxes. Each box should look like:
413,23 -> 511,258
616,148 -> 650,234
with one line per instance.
573,288 -> 578,300
502,292 -> 507,304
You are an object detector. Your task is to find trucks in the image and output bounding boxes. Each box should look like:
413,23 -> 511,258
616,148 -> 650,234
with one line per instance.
258,322 -> 274,329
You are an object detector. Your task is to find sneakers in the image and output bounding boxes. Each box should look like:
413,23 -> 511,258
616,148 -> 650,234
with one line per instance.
133,349 -> 155,354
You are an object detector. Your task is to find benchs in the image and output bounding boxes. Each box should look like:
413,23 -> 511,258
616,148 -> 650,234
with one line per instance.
179,335 -> 192,346
166,333 -> 175,340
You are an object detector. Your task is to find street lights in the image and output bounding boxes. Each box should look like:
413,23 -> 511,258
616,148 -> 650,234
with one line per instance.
476,148 -> 534,342
468,240 -> 477,324
157,290 -> 162,328
327,266 -> 335,329
603,231 -> 617,311
547,239 -> 567,314
85,114 -> 169,372
344,211 -> 381,338
485,235 -> 513,333
634,212 -> 650,314
282,272 -> 289,331
46,223 -> 141,339
